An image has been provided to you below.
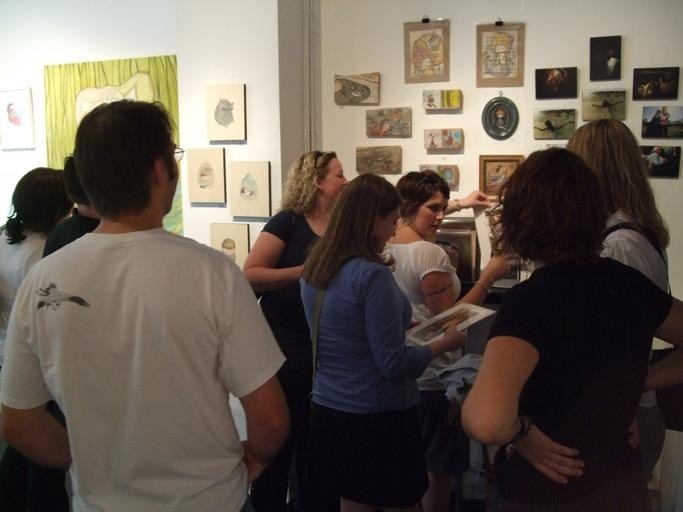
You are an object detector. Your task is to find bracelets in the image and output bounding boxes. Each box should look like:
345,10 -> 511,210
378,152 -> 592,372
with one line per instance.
473,280 -> 492,292
507,420 -> 532,442
453,195 -> 463,212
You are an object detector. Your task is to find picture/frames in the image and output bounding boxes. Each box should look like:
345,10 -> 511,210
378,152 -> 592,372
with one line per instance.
435,228 -> 476,284
479,154 -> 525,202
404,19 -> 450,84
440,217 -> 480,281
405,301 -> 496,345
476,22 -> 524,88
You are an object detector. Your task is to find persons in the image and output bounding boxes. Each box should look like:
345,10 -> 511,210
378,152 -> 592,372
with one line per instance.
0,100 -> 290,512
241,149 -> 348,512
660,107 -> 670,135
378,171 -> 514,322
534,121 -> 571,131
42,154 -> 101,428
460,147 -> 683,511
647,110 -> 661,137
590,98 -> 623,114
568,120 -> 668,511
299,173 -> 467,511
0,168 -> 84,512
644,147 -> 665,170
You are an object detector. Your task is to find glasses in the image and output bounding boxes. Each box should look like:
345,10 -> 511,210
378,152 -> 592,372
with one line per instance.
174,148 -> 185,161
